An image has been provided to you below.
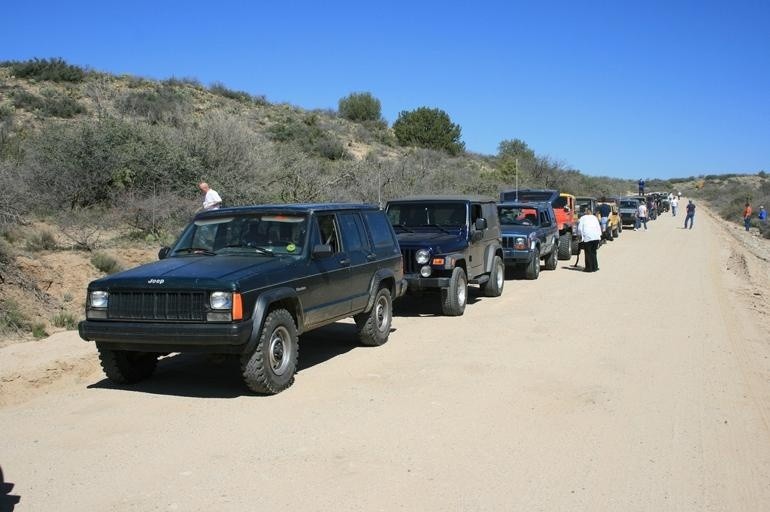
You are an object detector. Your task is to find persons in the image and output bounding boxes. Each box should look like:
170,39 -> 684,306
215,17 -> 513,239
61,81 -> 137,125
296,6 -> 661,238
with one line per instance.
195,183 -> 222,213
685,200 -> 695,229
577,178 -> 682,272
743,203 -> 752,231
758,205 -> 767,219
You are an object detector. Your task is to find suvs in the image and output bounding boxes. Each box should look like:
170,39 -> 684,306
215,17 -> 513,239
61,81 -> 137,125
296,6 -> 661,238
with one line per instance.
379,189 -> 670,317
77,197 -> 417,398
